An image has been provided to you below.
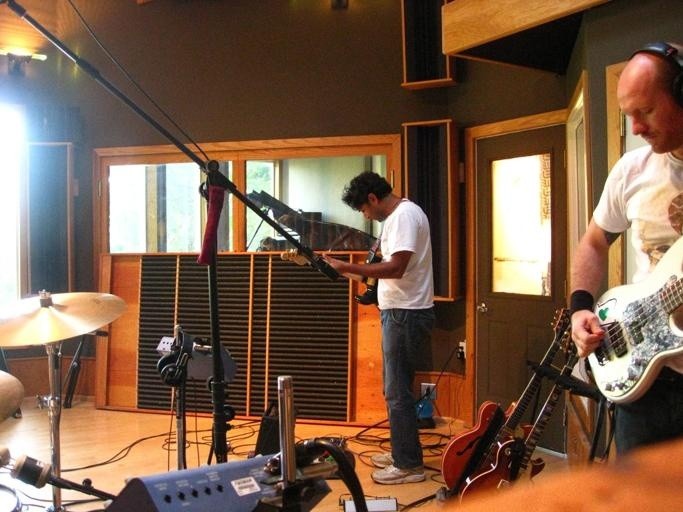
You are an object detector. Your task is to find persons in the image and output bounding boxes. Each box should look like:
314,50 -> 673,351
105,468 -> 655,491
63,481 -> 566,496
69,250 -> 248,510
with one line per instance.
569,42 -> 683,458
319,171 -> 435,484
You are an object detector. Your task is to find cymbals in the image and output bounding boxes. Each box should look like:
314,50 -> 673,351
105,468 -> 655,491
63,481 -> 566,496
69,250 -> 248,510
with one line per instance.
0,292 -> 127,348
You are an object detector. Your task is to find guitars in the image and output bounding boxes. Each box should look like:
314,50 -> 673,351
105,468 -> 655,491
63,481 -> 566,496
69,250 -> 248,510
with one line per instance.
281,247 -> 382,312
439,304 -> 573,495
585,232 -> 683,403
459,320 -> 578,503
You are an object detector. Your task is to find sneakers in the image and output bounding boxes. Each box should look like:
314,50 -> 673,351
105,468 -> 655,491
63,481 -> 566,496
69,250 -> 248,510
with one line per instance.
371,451 -> 425,485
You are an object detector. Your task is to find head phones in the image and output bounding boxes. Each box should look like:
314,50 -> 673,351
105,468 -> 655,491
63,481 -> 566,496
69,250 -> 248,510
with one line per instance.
626,42 -> 683,108
156,330 -> 194,388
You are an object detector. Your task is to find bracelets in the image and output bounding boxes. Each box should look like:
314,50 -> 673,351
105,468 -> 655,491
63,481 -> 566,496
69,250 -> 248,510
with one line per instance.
569,290 -> 594,315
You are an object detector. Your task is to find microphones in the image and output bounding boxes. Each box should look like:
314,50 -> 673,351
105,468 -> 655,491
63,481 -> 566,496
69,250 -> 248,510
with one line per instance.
300,245 -> 339,283
